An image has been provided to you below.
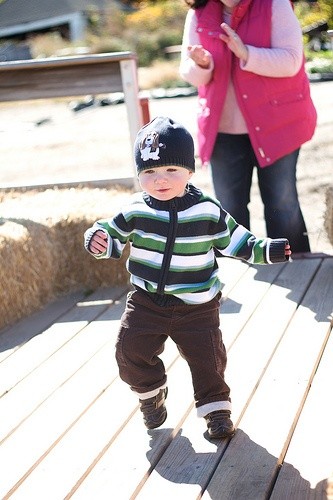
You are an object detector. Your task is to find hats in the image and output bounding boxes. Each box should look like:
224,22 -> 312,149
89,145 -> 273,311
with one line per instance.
134,117 -> 195,175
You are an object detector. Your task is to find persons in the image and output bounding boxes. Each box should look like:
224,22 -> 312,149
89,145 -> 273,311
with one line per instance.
84,115 -> 291,441
178,0 -> 317,251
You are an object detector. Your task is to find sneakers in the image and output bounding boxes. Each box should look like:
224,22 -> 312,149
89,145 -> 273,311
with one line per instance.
204,409 -> 235,437
140,387 -> 170,428
